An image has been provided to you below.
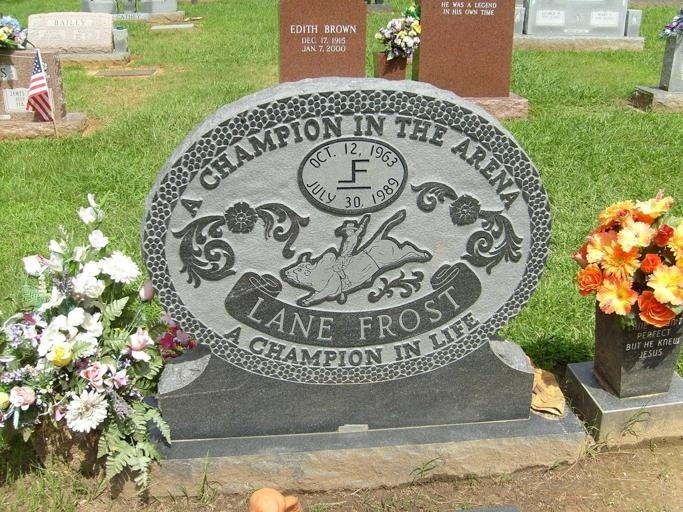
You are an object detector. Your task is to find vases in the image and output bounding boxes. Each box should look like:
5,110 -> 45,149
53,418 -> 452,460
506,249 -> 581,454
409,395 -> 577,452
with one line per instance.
374,52 -> 406,79
595,301 -> 683,399
31,420 -> 102,474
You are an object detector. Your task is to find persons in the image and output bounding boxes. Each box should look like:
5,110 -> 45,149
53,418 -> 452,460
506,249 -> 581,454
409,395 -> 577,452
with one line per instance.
335,213 -> 368,294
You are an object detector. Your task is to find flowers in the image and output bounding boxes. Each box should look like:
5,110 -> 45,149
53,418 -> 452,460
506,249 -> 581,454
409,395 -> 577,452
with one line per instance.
571,189 -> 683,327
0,17 -> 28,49
656,8 -> 683,40
378,0 -> 421,59
0,190 -> 196,487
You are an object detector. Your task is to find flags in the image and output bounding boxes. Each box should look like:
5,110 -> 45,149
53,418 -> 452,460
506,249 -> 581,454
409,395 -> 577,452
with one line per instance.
25,48 -> 54,122
